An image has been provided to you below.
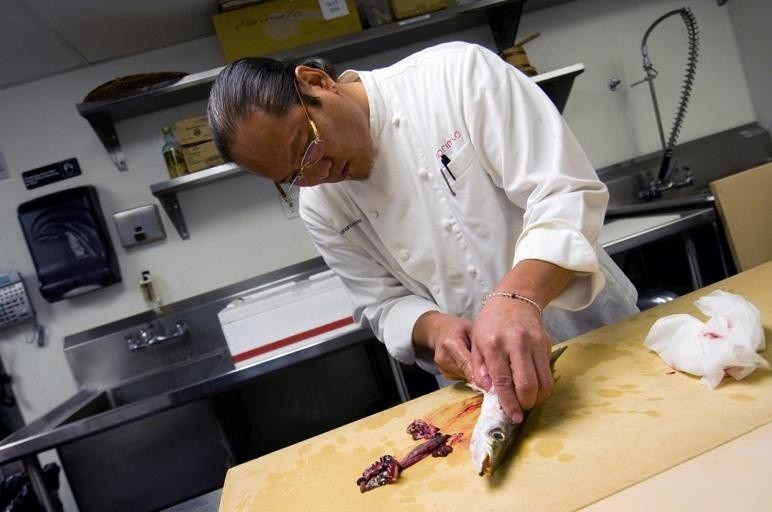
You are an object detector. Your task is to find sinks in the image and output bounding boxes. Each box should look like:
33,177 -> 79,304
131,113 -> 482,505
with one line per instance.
0,346 -> 256,512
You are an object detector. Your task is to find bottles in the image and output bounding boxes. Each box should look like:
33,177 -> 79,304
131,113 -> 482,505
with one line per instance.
161,123 -> 187,179
499,46 -> 536,77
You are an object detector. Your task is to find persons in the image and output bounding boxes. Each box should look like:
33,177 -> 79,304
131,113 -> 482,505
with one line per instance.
208,39 -> 640,424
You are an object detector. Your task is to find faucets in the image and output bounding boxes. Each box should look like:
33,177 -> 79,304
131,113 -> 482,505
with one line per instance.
123,320 -> 189,352
636,4 -> 702,202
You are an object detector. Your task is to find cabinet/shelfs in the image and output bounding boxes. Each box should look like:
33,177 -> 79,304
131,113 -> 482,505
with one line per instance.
76,0 -> 585,239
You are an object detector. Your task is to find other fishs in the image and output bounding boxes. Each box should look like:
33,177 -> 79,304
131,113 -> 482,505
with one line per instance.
464,341 -> 570,479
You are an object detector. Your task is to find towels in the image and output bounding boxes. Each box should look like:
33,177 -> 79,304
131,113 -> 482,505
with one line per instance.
644,290 -> 772,389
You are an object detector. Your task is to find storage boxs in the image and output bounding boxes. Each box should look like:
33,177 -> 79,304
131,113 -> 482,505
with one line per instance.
182,142 -> 225,173
175,116 -> 213,146
390,0 -> 447,20
209,0 -> 363,64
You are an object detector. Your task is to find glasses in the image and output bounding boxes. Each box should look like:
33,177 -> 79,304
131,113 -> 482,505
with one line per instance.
274,75 -> 327,211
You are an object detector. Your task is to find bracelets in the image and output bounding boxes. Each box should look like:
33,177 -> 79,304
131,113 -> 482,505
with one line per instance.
479,292 -> 544,316
